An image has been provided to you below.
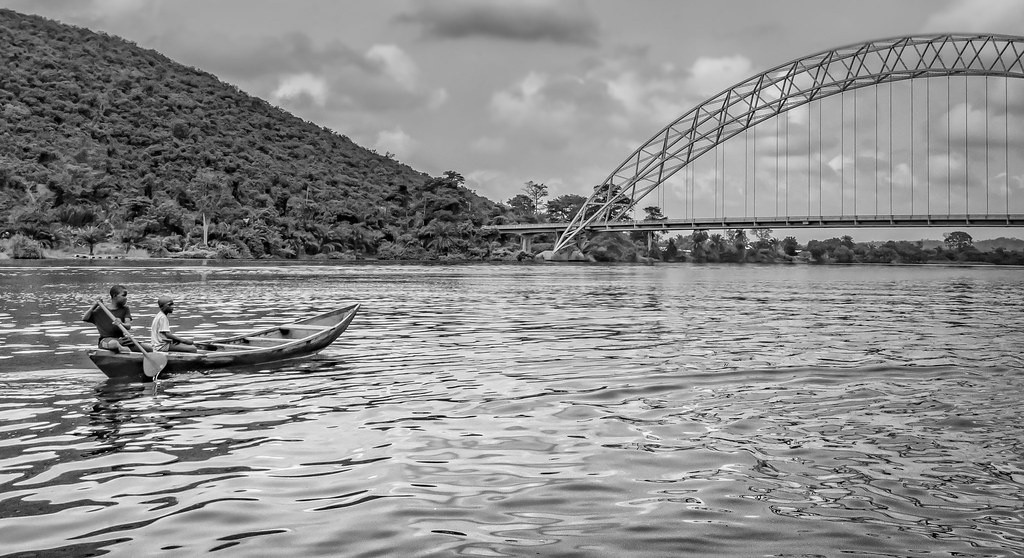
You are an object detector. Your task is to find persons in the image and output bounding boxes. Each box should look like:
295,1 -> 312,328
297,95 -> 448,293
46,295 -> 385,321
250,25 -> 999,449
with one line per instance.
81,285 -> 153,353
151,296 -> 197,353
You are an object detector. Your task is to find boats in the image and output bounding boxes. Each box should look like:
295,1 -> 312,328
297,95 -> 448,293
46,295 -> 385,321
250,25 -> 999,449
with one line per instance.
85,304 -> 362,381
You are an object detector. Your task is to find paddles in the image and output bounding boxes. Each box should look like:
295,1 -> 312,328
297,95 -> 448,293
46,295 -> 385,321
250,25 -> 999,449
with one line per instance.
96,300 -> 169,377
